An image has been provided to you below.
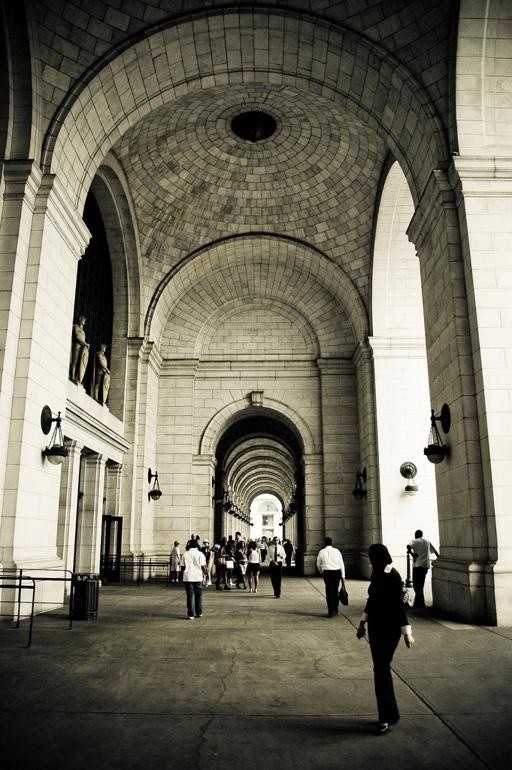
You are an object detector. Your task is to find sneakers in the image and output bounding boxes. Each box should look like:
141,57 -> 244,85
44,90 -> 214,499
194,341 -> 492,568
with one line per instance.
328,611 -> 338,617
244,586 -> 246,589
236,584 -> 241,588
196,614 -> 203,617
249,590 -> 253,592
172,579 -> 179,582
276,595 -> 280,598
189,616 -> 195,620
224,587 -> 231,590
216,587 -> 222,590
254,590 -> 258,593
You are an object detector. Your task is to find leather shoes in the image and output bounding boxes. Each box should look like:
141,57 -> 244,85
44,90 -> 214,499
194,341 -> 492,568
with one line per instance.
379,723 -> 390,733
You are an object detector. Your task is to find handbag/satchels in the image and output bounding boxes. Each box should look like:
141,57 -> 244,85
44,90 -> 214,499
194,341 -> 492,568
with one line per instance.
340,585 -> 349,605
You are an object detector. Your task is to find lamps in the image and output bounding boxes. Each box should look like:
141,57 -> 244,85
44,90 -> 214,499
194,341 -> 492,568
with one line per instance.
278,482 -> 299,527
353,467 -> 367,501
423,402 -> 451,464
148,467 -> 162,501
224,490 -> 253,526
399,462 -> 418,497
41,404 -> 71,465
249,390 -> 264,414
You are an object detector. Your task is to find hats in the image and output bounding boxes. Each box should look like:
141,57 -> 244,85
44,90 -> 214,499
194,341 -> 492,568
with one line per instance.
202,539 -> 210,544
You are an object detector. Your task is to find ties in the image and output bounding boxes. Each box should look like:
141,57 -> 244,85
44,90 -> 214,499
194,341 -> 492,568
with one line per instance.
275,547 -> 277,562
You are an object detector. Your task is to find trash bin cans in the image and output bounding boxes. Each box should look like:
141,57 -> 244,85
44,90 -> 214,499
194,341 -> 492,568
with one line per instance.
73,573 -> 99,620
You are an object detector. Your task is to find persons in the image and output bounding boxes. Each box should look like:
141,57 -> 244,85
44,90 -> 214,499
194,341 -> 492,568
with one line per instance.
406,531 -> 441,610
356,543 -> 416,736
179,539 -> 210,620
94,344 -> 111,403
167,532 -> 294,599
316,536 -> 347,617
71,315 -> 91,382
